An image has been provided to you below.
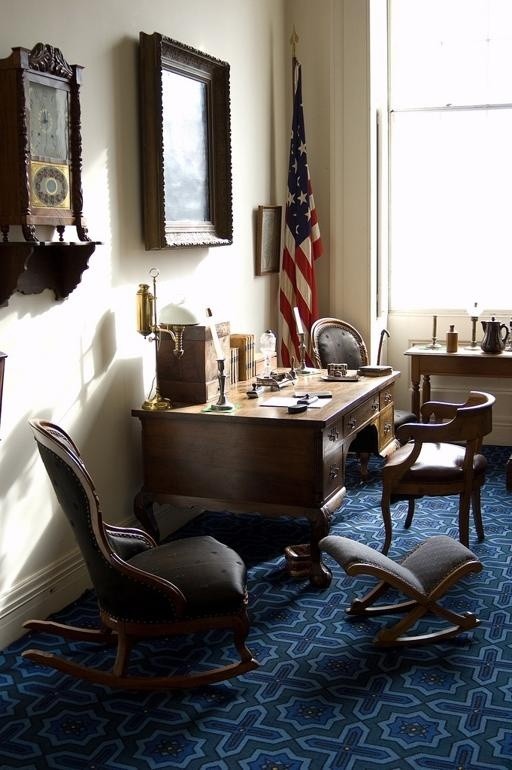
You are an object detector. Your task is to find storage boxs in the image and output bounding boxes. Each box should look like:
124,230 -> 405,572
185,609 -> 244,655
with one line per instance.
154,320 -> 231,404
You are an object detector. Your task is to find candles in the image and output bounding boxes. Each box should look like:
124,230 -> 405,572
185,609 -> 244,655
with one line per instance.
206,307 -> 225,361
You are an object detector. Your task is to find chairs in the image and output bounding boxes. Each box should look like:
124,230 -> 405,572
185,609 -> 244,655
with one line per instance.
20,417 -> 260,693
318,534 -> 484,649
381,390 -> 497,557
310,317 -> 417,461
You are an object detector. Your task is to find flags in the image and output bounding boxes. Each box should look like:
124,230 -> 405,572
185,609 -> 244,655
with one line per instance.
277,26 -> 322,367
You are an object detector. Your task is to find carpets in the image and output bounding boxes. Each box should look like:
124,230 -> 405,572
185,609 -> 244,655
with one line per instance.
0,444 -> 511,770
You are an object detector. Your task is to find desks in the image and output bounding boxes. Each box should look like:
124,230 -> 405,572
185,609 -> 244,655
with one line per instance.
403,342 -> 512,423
130,366 -> 402,590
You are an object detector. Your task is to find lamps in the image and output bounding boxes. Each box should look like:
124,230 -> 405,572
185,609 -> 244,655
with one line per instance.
136,266 -> 199,412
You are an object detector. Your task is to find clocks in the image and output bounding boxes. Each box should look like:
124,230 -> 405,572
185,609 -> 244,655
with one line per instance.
0,42 -> 103,308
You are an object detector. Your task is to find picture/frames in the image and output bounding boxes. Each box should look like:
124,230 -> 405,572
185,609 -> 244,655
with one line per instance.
137,30 -> 234,251
254,205 -> 281,276
